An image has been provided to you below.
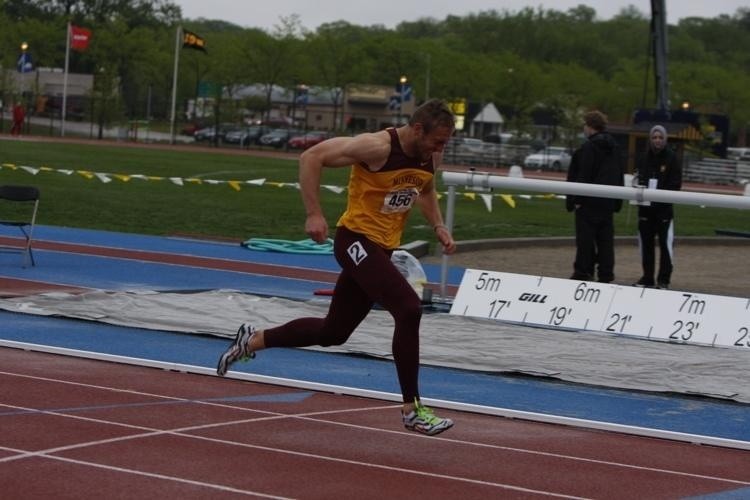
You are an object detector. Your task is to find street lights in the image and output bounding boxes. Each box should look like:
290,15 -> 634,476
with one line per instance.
17,41 -> 30,106
297,83 -> 308,131
398,76 -> 408,118
88,66 -> 107,140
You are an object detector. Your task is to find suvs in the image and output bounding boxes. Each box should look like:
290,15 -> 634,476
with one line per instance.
235,109 -> 262,126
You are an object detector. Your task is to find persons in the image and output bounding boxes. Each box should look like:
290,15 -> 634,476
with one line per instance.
10,101 -> 24,139
565,110 -> 625,283
217,99 -> 456,435
633,125 -> 682,290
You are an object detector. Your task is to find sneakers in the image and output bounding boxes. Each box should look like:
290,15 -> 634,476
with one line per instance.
399,404 -> 456,437
630,275 -> 669,289
215,320 -> 259,378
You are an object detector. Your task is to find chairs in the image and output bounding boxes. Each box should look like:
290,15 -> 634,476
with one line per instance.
0,184 -> 40,269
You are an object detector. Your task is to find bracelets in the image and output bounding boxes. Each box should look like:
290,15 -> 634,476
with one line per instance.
434,223 -> 449,230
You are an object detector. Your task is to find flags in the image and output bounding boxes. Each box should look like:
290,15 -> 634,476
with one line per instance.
18,50 -> 33,73
70,26 -> 91,49
182,28 -> 207,54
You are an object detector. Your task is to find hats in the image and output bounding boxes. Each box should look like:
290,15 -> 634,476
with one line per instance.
650,124 -> 669,146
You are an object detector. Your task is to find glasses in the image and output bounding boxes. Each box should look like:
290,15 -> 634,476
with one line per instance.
652,136 -> 664,140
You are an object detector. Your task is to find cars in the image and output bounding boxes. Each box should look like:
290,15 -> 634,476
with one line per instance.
193,121 -> 237,146
258,128 -> 304,151
225,124 -> 268,145
443,130 -> 571,172
289,130 -> 337,151
